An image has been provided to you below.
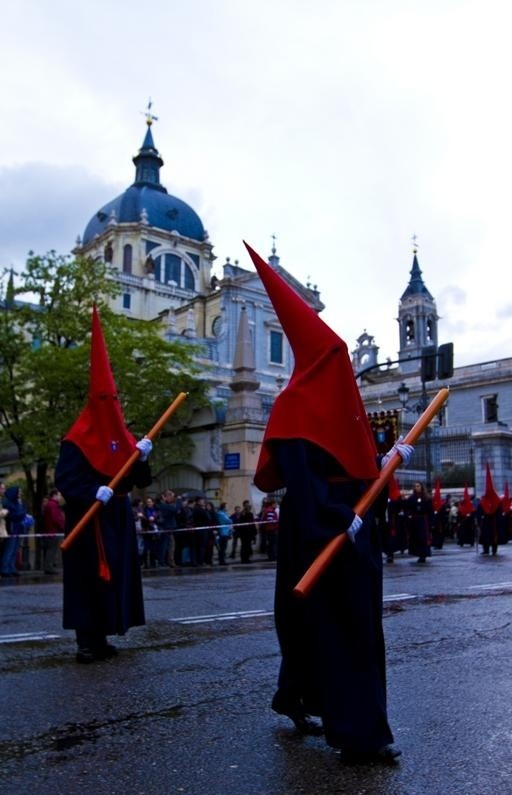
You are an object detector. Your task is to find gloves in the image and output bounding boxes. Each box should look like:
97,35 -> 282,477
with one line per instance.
96,486 -> 113,506
136,437 -> 154,464
381,435 -> 416,468
347,512 -> 364,545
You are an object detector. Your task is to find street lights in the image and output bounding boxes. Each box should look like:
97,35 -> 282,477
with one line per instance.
398,381 -> 449,497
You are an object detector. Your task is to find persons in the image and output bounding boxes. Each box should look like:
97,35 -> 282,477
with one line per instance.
242,240 -> 416,766
55,300 -> 156,665
383,461 -> 511,564
1,461 -> 66,576
132,488 -> 281,577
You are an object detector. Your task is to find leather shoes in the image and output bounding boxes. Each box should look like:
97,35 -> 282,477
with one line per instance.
94,633 -> 119,658
74,643 -> 94,663
340,745 -> 402,758
269,689 -> 326,736
